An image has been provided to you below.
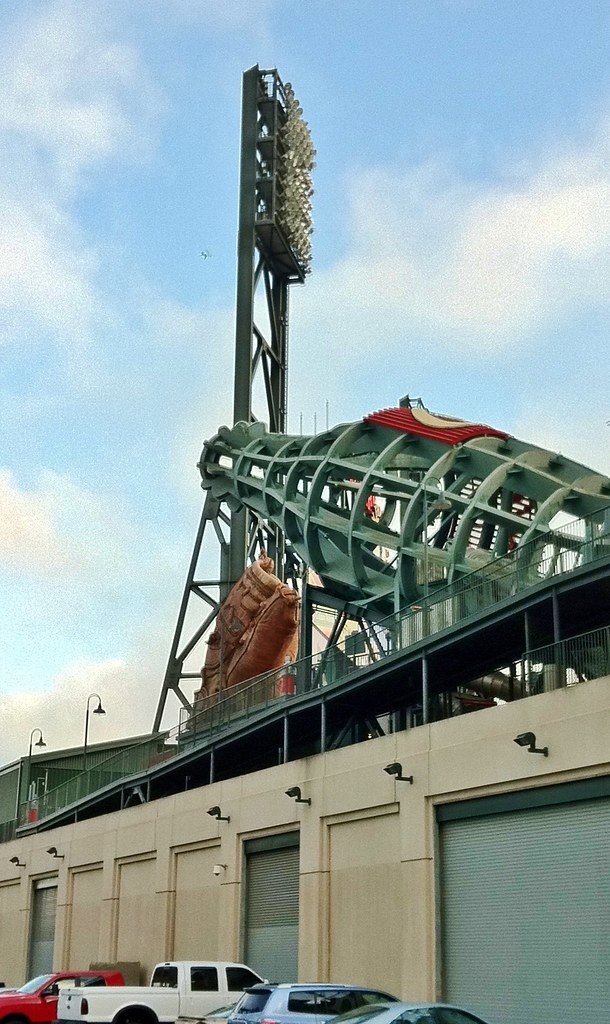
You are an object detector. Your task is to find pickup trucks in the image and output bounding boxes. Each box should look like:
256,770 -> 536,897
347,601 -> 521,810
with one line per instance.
57,959 -> 271,1024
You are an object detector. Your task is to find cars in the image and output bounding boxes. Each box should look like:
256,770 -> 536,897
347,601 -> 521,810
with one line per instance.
322,1001 -> 490,1024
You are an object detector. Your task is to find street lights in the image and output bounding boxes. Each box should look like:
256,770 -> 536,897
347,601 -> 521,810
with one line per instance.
26,728 -> 47,801
83,693 -> 107,770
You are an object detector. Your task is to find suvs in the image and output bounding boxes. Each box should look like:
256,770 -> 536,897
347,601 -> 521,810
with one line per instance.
227,981 -> 400,1024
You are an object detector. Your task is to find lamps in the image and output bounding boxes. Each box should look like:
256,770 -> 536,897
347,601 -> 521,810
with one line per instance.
284,786 -> 311,805
9,857 -> 26,867
383,762 -> 413,784
206,805 -> 230,823
47,847 -> 64,859
513,732 -> 547,757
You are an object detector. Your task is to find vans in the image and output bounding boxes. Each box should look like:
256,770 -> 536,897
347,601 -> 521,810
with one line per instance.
0,970 -> 127,1024
